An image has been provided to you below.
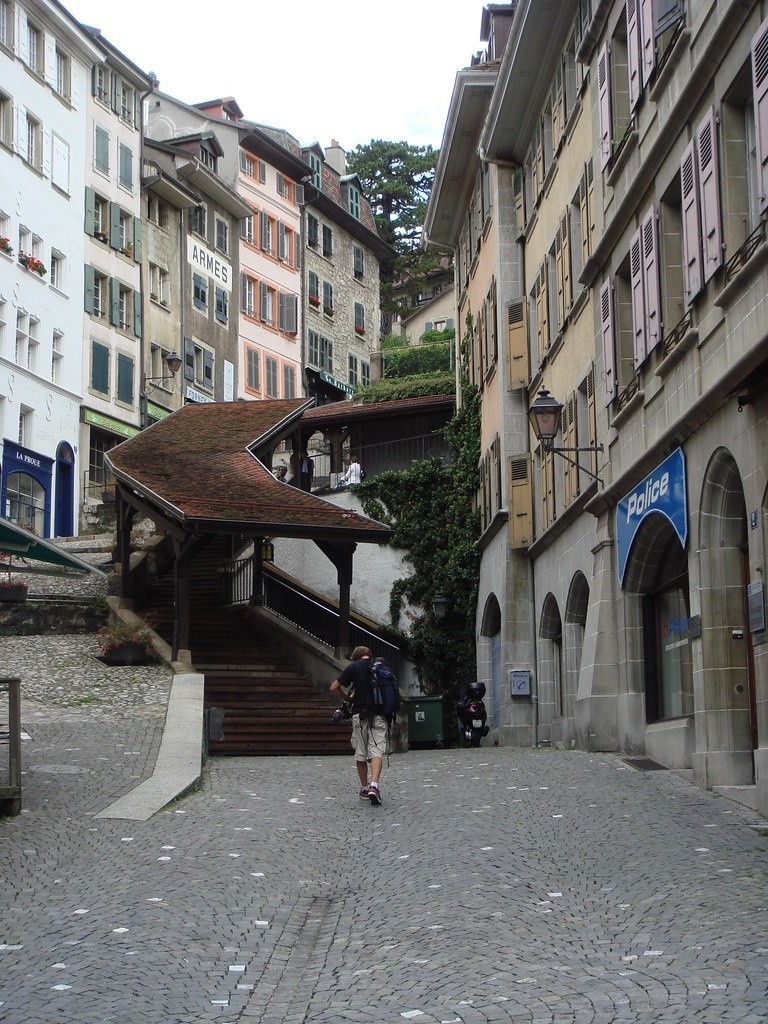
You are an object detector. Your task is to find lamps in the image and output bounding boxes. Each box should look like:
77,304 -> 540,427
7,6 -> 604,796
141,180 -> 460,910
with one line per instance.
431,591 -> 449,618
140,348 -> 182,398
262,536 -> 274,561
527,386 -> 604,485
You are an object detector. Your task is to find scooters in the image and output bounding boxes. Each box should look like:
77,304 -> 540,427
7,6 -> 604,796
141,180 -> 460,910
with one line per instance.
456,681 -> 488,748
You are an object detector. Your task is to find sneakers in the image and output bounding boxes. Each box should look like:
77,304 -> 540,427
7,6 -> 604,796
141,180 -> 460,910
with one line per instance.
358,786 -> 370,800
368,786 -> 382,805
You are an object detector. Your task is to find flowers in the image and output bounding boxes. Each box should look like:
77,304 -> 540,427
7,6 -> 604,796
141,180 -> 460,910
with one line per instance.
0,520 -> 38,589
0,235 -> 15,258
309,295 -> 321,308
355,325 -> 365,337
95,618 -> 167,666
18,249 -> 47,276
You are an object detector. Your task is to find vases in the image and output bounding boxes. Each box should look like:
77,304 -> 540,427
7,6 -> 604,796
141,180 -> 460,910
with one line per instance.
0,585 -> 28,602
108,643 -> 145,665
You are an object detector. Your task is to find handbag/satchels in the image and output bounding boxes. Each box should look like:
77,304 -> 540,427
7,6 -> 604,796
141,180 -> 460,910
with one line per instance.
360,467 -> 367,479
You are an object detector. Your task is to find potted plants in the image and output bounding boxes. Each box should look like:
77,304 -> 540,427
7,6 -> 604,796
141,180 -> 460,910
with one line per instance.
105,529 -> 143,562
125,241 -> 133,256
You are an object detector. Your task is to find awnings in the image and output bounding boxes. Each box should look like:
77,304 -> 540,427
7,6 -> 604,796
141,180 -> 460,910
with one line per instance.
304,365 -> 357,396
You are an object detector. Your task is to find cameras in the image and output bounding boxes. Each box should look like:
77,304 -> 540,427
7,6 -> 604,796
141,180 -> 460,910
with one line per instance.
332,709 -> 350,722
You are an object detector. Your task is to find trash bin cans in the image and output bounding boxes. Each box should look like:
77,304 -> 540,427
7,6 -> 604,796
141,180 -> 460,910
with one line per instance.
405,693 -> 448,749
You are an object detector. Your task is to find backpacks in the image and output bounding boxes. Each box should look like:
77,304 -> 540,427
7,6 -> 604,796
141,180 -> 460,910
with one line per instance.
368,656 -> 401,725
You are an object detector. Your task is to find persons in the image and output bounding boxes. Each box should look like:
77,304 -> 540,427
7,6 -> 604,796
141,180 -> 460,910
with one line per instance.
93,230 -> 108,245
301,450 -> 314,492
340,456 -> 361,485
330,646 -> 389,806
